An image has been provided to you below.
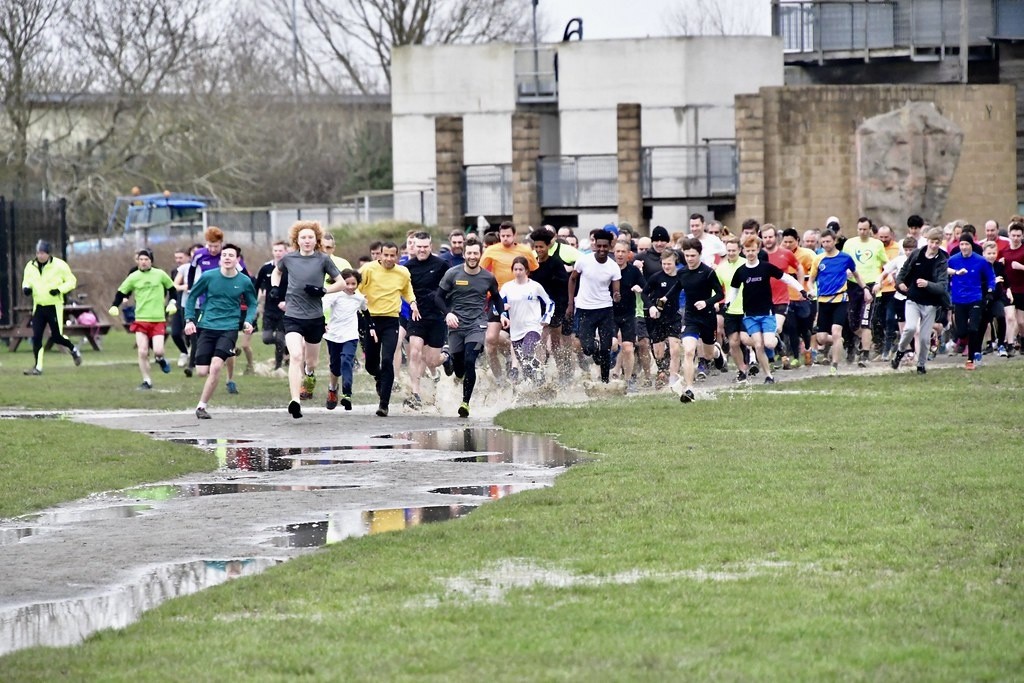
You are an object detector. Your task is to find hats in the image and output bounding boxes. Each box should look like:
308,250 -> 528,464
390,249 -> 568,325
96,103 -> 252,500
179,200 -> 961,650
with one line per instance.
650,226 -> 670,243
907,215 -> 924,228
139,248 -> 154,263
36,239 -> 49,254
960,234 -> 973,247
604,224 -> 619,239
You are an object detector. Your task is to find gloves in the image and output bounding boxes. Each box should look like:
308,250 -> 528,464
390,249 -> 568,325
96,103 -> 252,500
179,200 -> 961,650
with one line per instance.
24,287 -> 32,296
49,289 -> 60,296
108,306 -> 119,316
303,284 -> 327,298
165,299 -> 177,315
269,285 -> 279,299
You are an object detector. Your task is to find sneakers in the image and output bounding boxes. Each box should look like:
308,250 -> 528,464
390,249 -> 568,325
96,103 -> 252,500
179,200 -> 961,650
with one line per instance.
156,357 -> 170,373
137,381 -> 152,391
196,408 -> 211,419
24,368 -> 42,375
70,349 -> 81,366
226,382 -> 238,394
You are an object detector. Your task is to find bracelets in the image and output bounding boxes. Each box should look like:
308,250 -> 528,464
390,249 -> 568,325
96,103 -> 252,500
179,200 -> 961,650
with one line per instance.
863,286 -> 870,290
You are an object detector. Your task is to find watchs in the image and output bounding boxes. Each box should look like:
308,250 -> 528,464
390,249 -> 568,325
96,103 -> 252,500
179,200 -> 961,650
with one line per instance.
185,319 -> 193,323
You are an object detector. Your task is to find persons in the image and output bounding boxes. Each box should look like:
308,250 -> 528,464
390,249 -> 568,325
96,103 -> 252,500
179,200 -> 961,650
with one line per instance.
171,227 -> 293,395
269,221 -> 346,419
108,248 -> 177,390
21,239 -> 82,376
184,244 -> 258,419
300,213 -> 1024,414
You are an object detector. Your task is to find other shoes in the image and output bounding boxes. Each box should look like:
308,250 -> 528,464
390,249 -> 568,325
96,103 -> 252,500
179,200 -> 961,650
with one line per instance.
280,335 -> 1024,419
184,369 -> 193,377
188,346 -> 192,357
178,352 -> 187,367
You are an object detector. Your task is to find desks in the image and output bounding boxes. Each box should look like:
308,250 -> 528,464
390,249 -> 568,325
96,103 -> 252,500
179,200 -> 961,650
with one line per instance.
8,305 -> 104,353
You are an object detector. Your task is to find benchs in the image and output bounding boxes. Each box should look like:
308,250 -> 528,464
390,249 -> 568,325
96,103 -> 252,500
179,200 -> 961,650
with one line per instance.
0,324 -> 10,329
64,324 -> 113,328
